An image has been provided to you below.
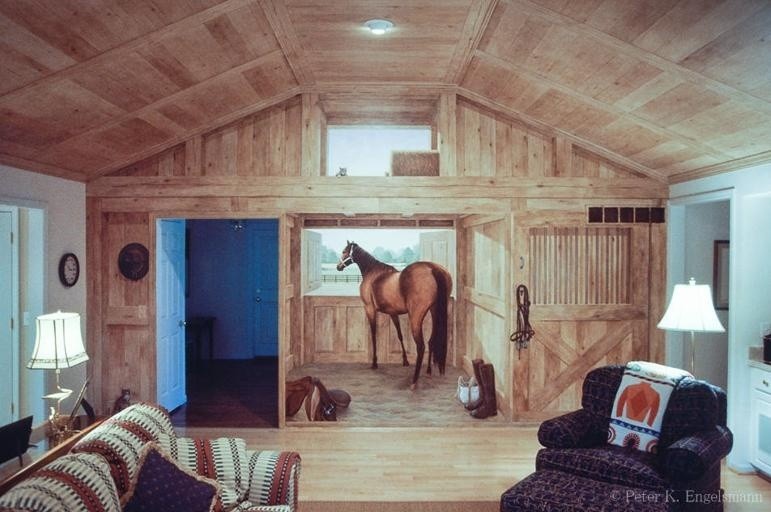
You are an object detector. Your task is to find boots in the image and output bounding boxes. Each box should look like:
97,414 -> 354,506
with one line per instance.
457,359 -> 497,418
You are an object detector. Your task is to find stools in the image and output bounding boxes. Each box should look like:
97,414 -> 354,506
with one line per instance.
496,469 -> 681,512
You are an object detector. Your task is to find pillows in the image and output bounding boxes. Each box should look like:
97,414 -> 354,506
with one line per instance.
118,441 -> 220,511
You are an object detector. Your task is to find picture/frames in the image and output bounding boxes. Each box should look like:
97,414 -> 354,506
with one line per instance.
65,378 -> 95,423
713,239 -> 730,311
0,414 -> 38,470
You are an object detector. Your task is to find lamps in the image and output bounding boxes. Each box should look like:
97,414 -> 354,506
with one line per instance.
654,275 -> 726,374
23,308 -> 90,441
362,18 -> 394,35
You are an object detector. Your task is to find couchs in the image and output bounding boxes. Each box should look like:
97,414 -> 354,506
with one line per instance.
1,402 -> 303,512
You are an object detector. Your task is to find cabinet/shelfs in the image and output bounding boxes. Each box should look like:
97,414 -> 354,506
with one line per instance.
749,365 -> 770,481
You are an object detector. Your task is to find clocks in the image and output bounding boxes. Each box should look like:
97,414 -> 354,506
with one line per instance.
58,252 -> 79,288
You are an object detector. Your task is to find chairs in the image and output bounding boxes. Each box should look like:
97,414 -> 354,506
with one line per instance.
533,360 -> 734,512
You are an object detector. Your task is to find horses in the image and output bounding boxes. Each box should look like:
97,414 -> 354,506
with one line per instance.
337,240 -> 452,391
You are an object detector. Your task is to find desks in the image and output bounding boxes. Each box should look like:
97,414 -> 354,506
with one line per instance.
1,413 -> 112,495
185,316 -> 217,384
